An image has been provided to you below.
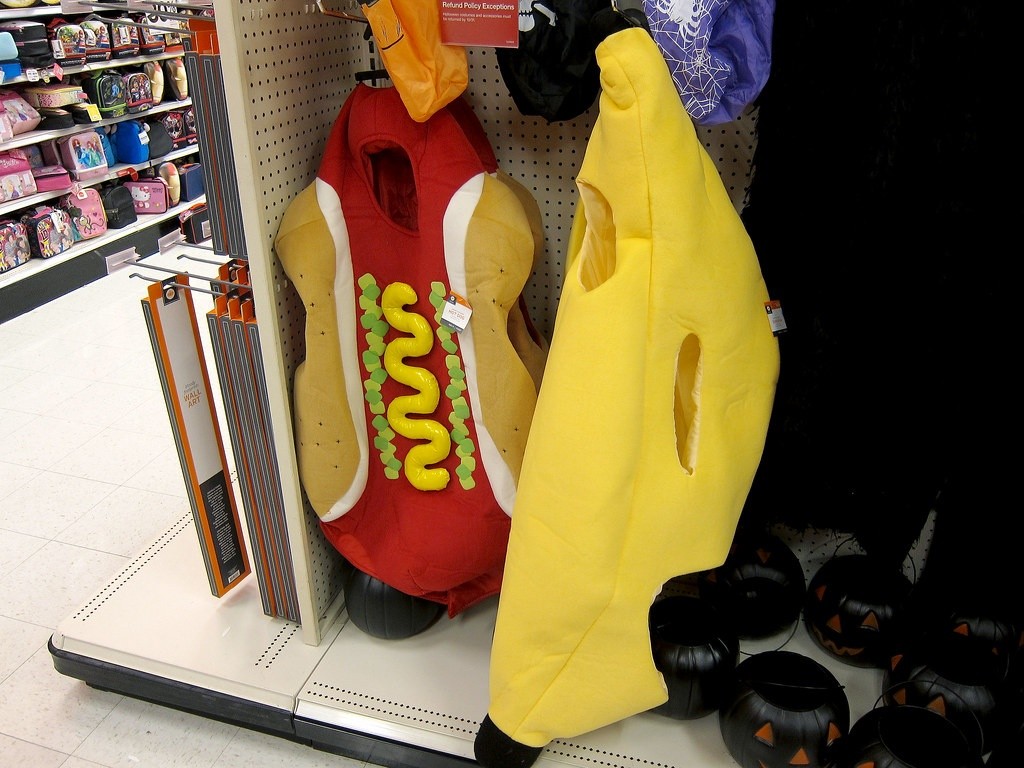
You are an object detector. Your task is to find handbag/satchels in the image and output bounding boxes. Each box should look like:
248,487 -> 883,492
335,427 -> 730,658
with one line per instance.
179,203 -> 212,244
179,163 -> 205,201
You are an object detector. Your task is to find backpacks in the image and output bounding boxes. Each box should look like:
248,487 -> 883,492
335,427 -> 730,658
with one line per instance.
0,8 -> 205,274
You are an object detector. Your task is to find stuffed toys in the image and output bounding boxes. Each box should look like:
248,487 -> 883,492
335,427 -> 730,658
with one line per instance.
472,11 -> 779,768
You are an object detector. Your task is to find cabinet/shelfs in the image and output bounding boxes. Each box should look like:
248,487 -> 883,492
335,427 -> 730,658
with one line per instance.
0,0 -> 209,325
